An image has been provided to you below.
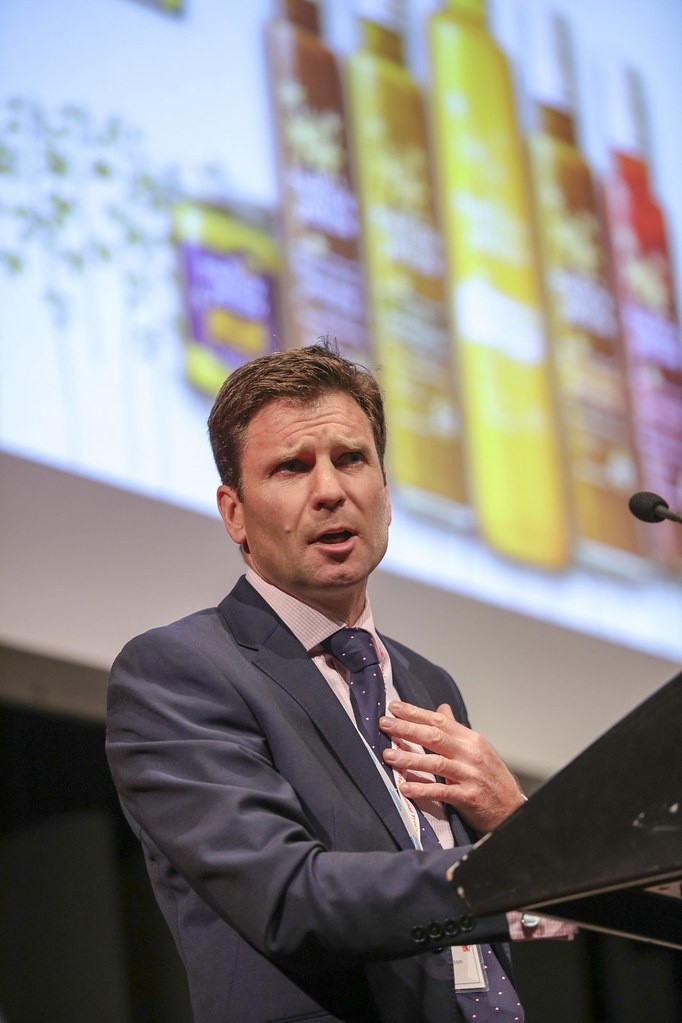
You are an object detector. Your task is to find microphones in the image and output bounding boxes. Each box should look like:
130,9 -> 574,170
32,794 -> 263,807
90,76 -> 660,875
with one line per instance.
629,492 -> 682,524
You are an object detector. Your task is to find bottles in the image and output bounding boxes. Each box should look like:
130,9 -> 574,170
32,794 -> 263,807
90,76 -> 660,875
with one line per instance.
170,0 -> 682,578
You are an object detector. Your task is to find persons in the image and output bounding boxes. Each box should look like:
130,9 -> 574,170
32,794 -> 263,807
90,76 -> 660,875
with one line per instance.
104,343 -> 578,1023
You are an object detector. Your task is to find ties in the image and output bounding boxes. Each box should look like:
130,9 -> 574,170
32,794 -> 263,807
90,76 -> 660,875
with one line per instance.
321,629 -> 524,1023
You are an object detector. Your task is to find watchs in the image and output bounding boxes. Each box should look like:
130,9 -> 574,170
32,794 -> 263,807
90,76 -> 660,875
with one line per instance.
520,913 -> 541,938
